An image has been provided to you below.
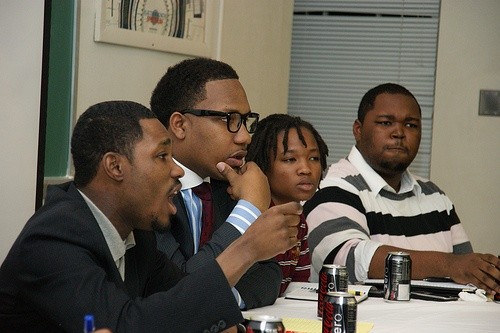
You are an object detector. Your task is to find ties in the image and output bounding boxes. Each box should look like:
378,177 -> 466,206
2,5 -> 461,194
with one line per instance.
191,182 -> 215,251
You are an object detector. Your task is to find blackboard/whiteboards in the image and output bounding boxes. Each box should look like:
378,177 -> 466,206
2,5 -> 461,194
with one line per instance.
45,0 -> 78,184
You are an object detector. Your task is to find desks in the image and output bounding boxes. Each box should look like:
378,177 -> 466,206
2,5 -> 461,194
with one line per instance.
241,277 -> 500,333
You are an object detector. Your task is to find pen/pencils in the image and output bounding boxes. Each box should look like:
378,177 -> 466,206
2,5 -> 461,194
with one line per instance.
84,315 -> 95,333
308,290 -> 365,297
424,277 -> 455,282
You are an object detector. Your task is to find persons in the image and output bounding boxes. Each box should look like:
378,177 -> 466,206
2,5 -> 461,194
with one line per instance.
1,100 -> 305,333
300,83 -> 500,297
141,56 -> 282,312
244,113 -> 328,298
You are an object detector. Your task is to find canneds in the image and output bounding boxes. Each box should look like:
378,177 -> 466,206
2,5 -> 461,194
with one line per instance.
246,315 -> 285,333
384,251 -> 412,303
322,292 -> 357,333
317,264 -> 349,320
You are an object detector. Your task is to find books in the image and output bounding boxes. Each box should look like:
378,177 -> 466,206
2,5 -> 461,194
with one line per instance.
285,278 -> 488,307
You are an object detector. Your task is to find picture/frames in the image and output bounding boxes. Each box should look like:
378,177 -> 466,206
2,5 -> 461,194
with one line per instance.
94,0 -> 223,60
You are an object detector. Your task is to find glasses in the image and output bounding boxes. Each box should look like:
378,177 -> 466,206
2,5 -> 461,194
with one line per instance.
180,109 -> 259,134
